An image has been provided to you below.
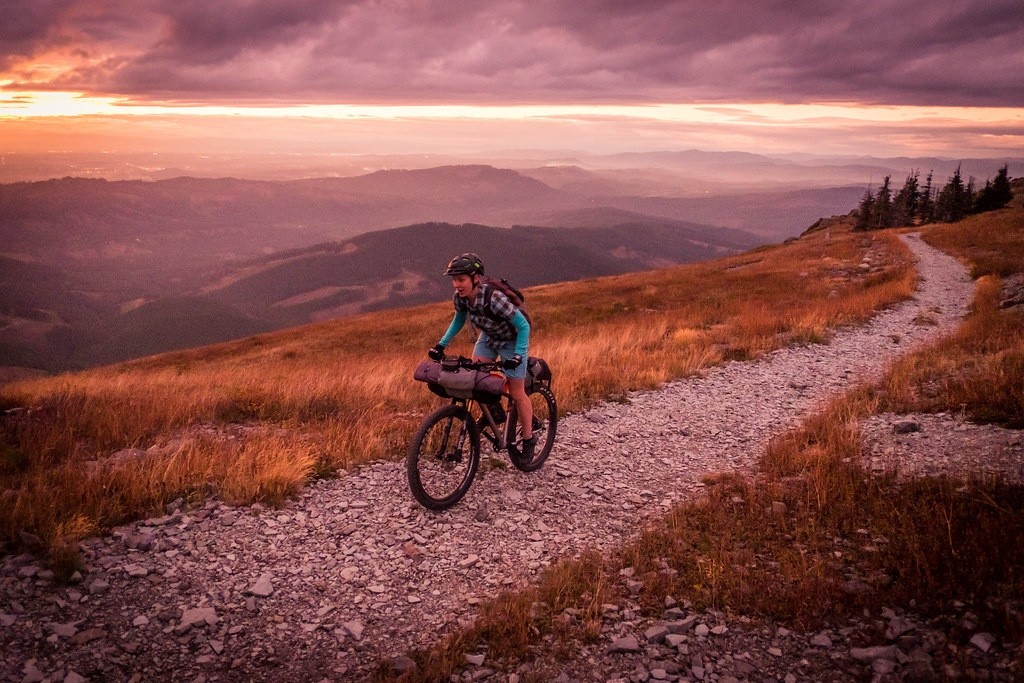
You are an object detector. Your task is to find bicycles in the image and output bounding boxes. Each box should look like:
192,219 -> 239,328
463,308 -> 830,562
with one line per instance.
408,354 -> 558,510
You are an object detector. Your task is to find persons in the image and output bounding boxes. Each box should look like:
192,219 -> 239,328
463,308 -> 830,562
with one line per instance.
428,253 -> 537,466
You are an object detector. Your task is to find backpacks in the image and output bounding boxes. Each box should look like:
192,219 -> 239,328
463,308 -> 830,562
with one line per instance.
456,276 -> 524,322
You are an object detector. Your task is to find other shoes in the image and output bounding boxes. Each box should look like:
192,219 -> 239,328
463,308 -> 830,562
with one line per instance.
519,433 -> 538,465
474,403 -> 507,433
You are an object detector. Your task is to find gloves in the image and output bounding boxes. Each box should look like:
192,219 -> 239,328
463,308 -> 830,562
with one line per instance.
505,354 -> 522,370
428,343 -> 445,361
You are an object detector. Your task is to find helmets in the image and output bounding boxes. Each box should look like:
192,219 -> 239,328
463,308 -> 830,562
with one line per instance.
443,252 -> 485,277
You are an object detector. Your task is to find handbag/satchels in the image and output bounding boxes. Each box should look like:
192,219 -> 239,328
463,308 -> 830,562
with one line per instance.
527,356 -> 552,381
